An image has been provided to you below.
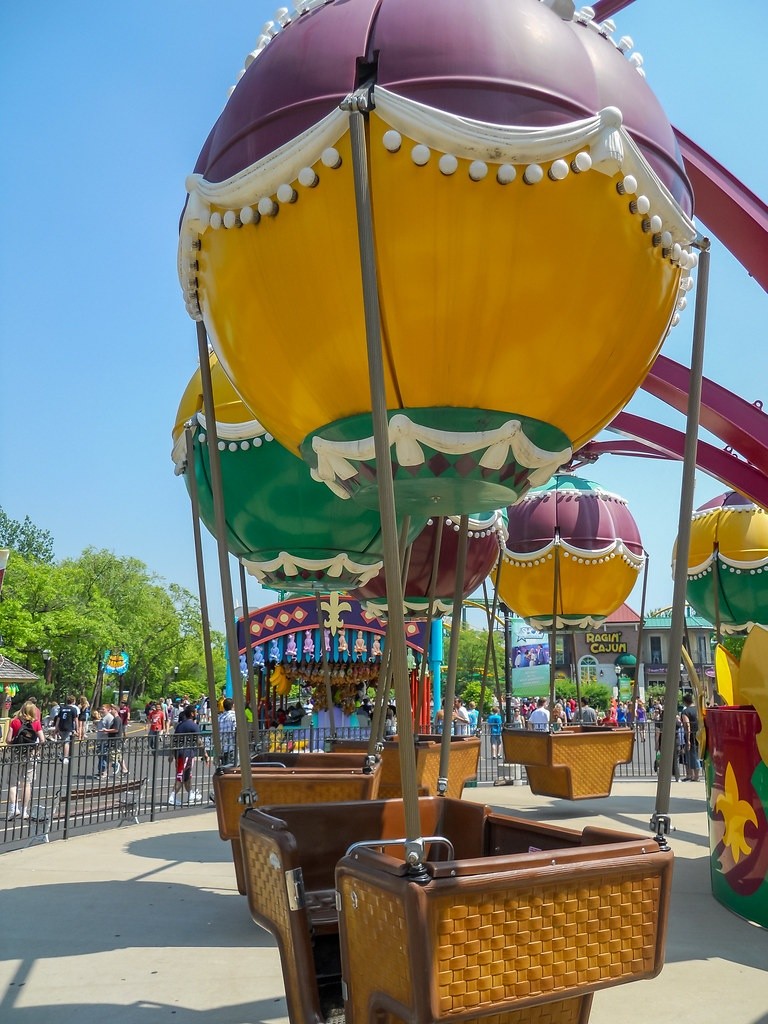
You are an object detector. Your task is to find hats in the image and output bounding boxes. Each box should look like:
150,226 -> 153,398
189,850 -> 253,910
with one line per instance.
111,704 -> 120,712
155,704 -> 161,710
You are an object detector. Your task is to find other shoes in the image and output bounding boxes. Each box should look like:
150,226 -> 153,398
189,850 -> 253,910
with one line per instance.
493,757 -> 495,760
691,778 -> 700,782
498,755 -> 503,759
682,777 -> 692,782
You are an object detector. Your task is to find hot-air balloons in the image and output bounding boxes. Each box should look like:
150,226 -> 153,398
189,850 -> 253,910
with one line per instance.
480,470 -> 650,800
180,1 -> 706,1024
327,507 -> 503,802
170,354 -> 428,901
670,480 -> 767,931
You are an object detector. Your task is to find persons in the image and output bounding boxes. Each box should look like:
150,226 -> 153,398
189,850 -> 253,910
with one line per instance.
0,694 -> 238,822
433,693 -> 707,782
264,698 -> 397,740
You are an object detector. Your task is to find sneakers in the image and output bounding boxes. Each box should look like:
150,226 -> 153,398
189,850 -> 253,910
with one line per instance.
5,809 -> 21,822
187,794 -> 202,803
168,797 -> 181,806
111,762 -> 120,775
117,769 -> 131,779
91,772 -> 109,780
21,814 -> 30,820
63,758 -> 69,764
123,739 -> 128,745
210,792 -> 216,804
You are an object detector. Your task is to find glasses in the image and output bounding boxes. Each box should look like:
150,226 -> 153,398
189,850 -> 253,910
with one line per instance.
515,711 -> 520,713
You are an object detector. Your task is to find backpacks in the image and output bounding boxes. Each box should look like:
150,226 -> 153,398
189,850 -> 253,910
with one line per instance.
12,717 -> 37,754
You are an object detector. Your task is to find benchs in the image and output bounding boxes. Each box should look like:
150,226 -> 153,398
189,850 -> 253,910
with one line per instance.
5,777 -> 149,847
